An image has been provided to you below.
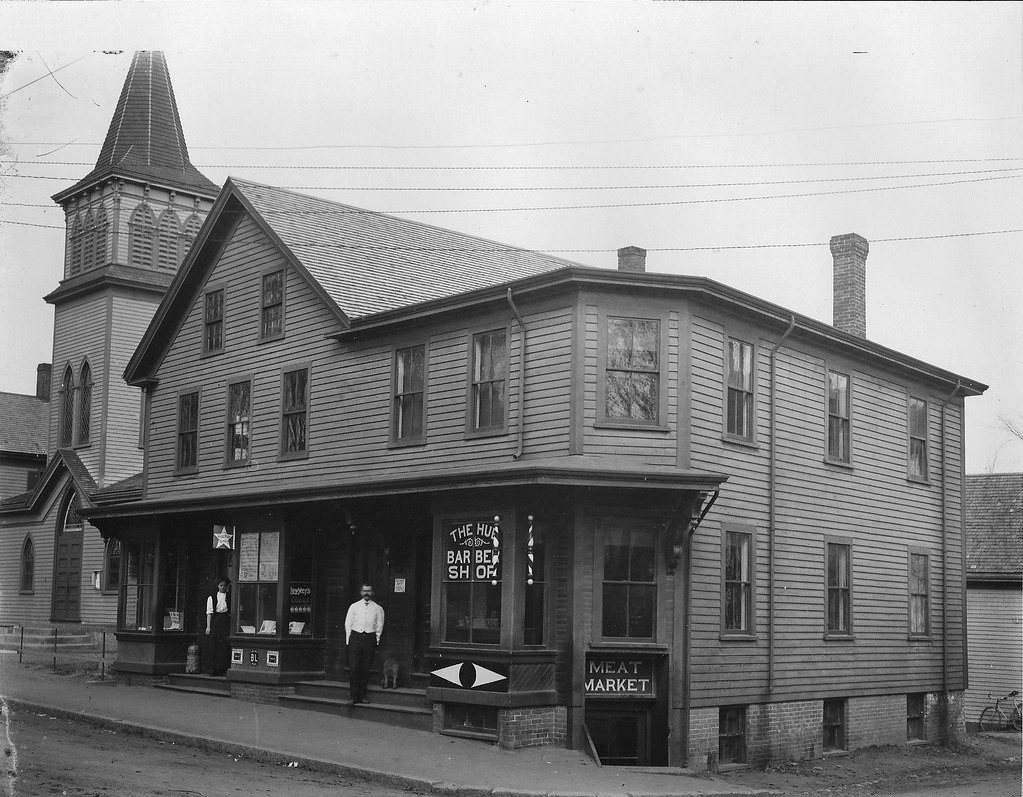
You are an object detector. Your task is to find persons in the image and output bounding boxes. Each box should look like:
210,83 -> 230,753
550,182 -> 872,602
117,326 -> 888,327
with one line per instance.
344,583 -> 385,706
205,576 -> 231,677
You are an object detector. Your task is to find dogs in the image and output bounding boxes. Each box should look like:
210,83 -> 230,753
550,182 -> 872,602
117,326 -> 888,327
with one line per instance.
382,658 -> 400,689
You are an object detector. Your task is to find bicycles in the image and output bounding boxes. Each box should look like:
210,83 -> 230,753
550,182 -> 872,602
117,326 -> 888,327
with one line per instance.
979,690 -> 1023,733
